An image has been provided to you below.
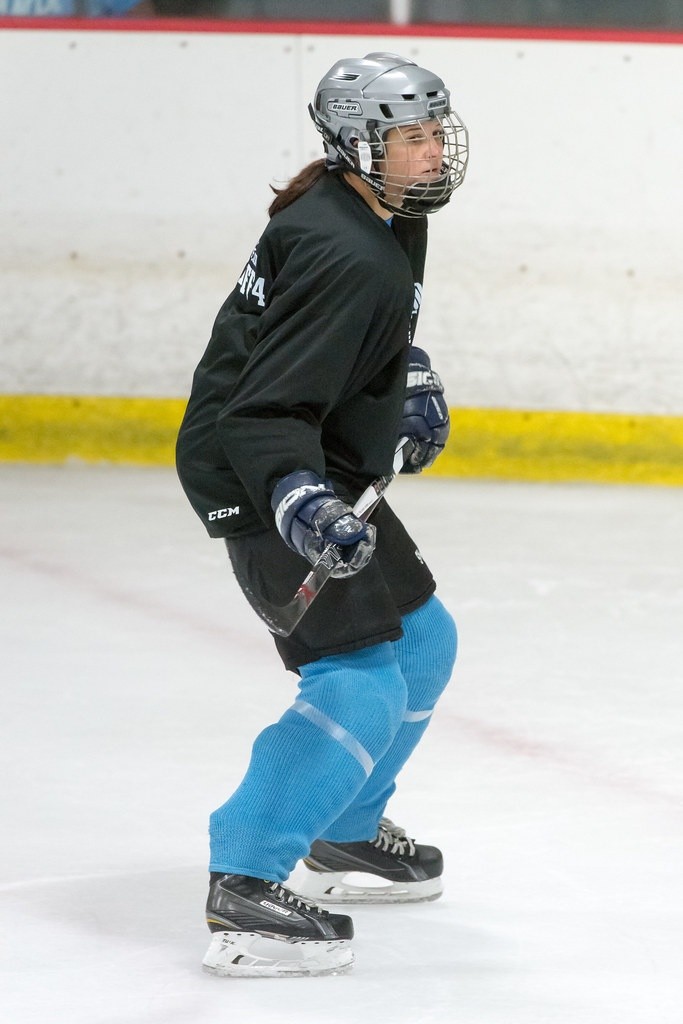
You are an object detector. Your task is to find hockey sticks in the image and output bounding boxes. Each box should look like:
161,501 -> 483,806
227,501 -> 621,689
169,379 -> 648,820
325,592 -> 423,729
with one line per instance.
238,437 -> 415,640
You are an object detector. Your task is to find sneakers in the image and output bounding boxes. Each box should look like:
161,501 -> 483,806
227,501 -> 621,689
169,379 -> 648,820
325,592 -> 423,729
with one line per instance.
301,826 -> 445,903
203,868 -> 354,980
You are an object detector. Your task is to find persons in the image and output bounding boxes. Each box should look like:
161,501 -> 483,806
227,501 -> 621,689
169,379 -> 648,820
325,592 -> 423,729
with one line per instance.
172,52 -> 464,975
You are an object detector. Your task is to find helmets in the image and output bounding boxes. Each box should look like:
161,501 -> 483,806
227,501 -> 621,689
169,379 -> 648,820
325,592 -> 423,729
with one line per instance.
311,53 -> 469,216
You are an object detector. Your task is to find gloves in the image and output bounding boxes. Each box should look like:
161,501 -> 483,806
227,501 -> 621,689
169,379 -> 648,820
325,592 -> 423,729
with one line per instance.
394,346 -> 449,474
271,471 -> 376,583
